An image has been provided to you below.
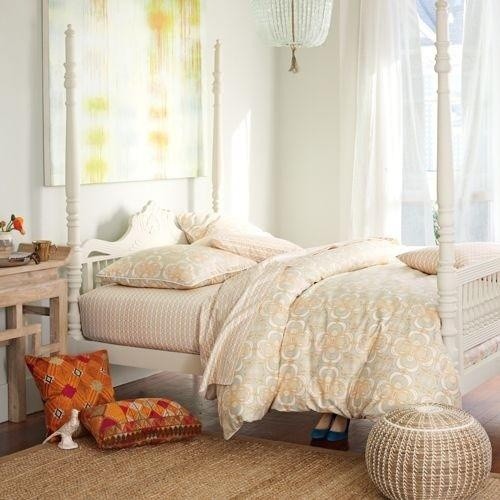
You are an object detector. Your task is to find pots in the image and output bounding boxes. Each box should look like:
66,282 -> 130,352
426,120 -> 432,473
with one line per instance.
0,251 -> 40,266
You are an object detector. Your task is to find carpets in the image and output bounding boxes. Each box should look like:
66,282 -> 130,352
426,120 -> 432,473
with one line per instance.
0,430 -> 500,500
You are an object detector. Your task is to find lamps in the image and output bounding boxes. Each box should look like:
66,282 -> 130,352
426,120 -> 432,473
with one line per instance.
246,0 -> 332,74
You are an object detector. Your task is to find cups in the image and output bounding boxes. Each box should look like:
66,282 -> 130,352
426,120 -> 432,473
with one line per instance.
32,239 -> 57,262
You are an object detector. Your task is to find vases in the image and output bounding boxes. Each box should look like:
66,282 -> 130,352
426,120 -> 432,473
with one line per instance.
0,231 -> 12,241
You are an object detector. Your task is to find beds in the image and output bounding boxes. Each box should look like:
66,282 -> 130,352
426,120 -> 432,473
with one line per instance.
62,0 -> 500,443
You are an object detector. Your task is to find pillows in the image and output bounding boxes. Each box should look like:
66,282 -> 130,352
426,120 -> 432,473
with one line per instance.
79,395 -> 201,450
94,243 -> 258,289
176,211 -> 303,263
396,240 -> 494,276
26,349 -> 116,442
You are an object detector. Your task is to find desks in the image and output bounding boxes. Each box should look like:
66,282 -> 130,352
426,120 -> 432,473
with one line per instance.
0,241 -> 72,424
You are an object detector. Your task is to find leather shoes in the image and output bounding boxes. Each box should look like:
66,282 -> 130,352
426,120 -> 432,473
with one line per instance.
309,412 -> 337,440
326,413 -> 352,442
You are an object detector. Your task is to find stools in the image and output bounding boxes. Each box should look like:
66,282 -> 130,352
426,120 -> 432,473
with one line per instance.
365,402 -> 493,500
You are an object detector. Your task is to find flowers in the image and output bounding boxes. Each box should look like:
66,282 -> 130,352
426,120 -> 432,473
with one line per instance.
0,214 -> 24,235
